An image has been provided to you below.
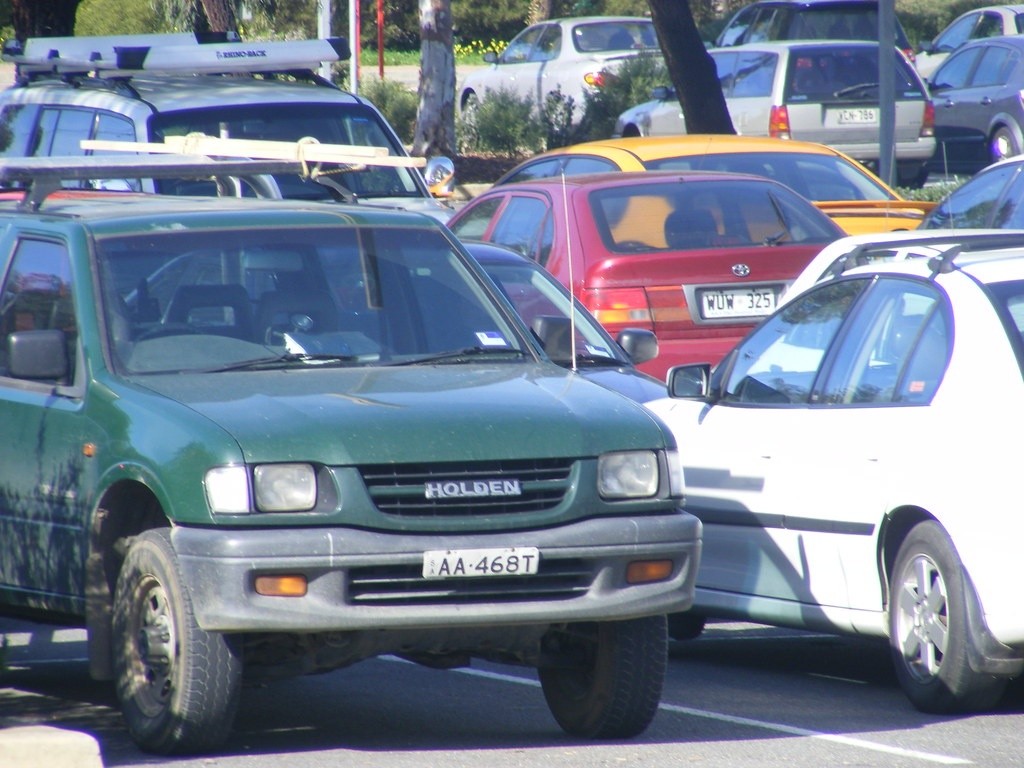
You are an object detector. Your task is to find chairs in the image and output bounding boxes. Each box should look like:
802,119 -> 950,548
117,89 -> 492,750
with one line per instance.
662,208 -> 717,254
255,287 -> 338,342
160,282 -> 254,344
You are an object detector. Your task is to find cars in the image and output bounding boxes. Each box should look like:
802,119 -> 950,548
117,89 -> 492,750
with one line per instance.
725,227 -> 1024,412
920,36 -> 1023,182
270,240 -> 677,418
703,1 -> 920,104
911,5 -> 1024,90
610,38 -> 938,191
611,234 -> 1024,719
421,169 -> 882,394
457,16 -> 671,139
491,129 -> 944,265
913,148 -> 1024,344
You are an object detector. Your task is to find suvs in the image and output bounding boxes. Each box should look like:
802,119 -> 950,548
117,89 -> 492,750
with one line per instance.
0,144 -> 702,757
1,25 -> 464,301
